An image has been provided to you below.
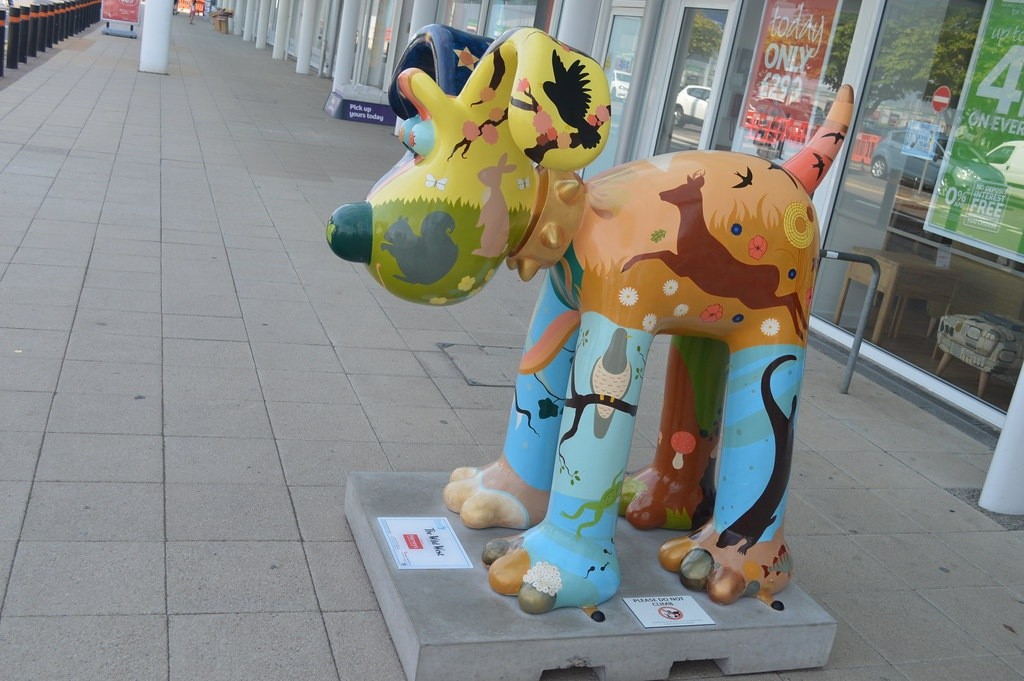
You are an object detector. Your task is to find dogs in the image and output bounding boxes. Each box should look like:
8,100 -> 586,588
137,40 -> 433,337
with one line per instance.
324,21 -> 856,615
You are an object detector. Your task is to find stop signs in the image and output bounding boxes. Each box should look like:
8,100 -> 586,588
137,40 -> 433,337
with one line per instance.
932,86 -> 951,113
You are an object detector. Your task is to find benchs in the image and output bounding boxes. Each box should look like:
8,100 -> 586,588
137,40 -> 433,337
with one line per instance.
936,314 -> 1024,398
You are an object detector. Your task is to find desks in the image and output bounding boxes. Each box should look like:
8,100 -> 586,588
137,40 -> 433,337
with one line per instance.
832,244 -> 963,346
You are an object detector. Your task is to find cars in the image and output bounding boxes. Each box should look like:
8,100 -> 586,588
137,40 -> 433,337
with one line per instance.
605,70 -> 632,102
870,128 -> 1008,208
672,84 -> 712,128
971,138 -> 1024,206
749,95 -> 898,147
